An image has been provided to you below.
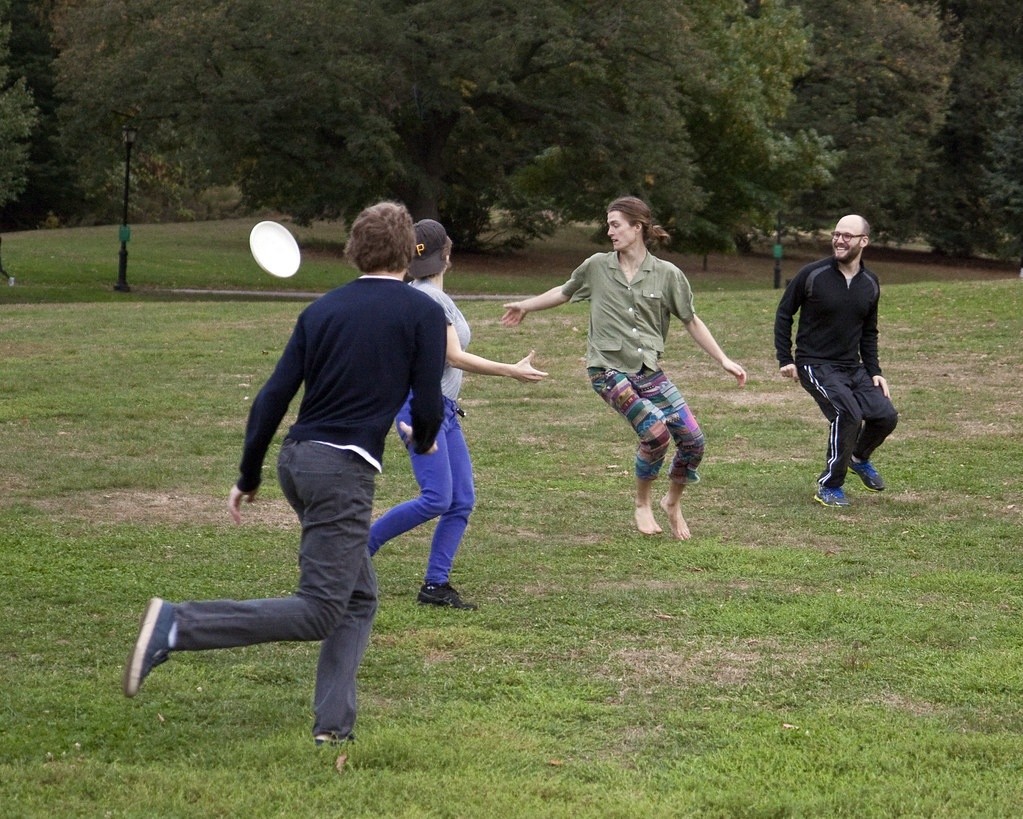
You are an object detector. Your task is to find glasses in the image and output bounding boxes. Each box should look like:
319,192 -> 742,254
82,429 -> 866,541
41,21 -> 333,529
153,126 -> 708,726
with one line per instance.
832,231 -> 866,241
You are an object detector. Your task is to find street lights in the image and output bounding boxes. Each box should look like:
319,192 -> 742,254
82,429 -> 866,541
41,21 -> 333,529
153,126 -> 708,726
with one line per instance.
112,116 -> 141,297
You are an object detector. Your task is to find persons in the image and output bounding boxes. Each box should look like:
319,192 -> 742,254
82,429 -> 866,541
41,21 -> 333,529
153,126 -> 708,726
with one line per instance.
122,203 -> 450,747
0,237 -> 15,287
501,196 -> 748,539
774,214 -> 898,507
368,219 -> 547,611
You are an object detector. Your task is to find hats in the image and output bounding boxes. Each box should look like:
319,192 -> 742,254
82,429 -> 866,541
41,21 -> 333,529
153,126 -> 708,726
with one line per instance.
409,220 -> 446,279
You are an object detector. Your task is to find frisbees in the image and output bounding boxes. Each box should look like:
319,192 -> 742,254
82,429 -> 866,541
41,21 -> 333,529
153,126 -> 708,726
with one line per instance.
250,220 -> 300,279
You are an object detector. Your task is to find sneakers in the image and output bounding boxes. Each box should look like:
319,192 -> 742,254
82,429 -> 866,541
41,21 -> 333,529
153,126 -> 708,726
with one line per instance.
417,585 -> 478,611
847,457 -> 885,493
814,485 -> 852,507
123,597 -> 175,698
315,737 -> 360,748
